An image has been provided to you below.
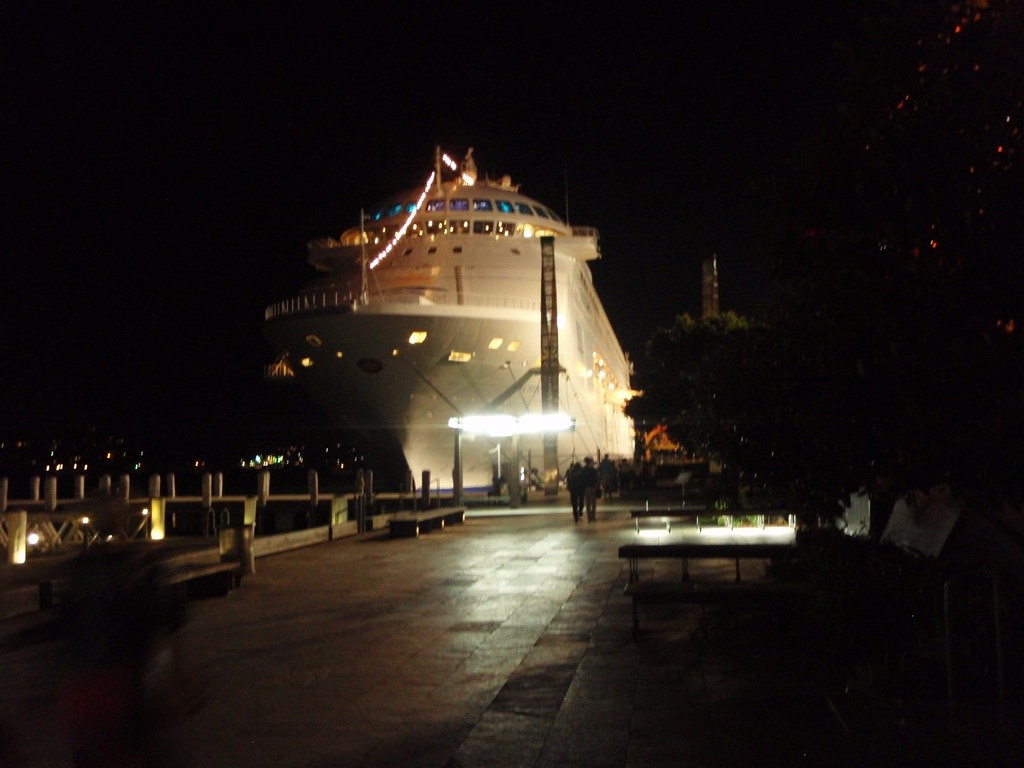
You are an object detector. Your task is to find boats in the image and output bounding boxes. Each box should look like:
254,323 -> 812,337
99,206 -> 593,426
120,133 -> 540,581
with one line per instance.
259,138 -> 638,493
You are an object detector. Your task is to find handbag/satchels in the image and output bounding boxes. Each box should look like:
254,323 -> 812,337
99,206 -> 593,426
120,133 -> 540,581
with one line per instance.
596,485 -> 603,498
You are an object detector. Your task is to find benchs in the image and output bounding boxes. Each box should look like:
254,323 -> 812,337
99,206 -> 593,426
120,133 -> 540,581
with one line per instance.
628,506 -> 792,529
622,582 -> 772,643
619,543 -> 797,579
169,559 -> 245,601
387,506 -> 466,538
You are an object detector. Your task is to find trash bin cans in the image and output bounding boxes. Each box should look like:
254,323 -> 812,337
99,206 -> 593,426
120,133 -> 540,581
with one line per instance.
218,521 -> 259,576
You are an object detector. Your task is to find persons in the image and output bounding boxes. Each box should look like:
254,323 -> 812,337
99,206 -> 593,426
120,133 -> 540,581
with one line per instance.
566,454 -> 629,521
47,507 -> 207,768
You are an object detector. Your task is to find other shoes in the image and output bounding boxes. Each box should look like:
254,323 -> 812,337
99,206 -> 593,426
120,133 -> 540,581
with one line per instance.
575,519 -> 578,524
588,517 -> 592,522
593,518 -> 597,522
